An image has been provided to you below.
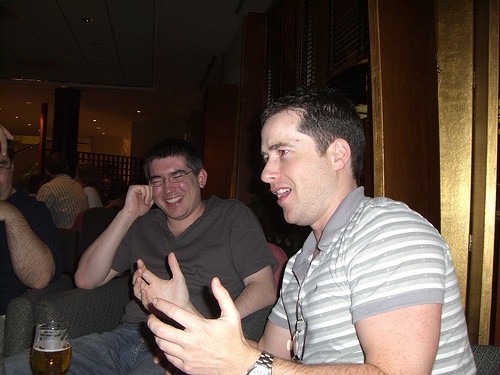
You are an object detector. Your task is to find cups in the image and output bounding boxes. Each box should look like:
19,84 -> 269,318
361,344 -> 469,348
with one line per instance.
31,322 -> 72,375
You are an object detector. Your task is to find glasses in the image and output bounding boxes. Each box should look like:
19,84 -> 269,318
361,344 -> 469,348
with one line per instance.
148,170 -> 193,187
291,299 -> 308,364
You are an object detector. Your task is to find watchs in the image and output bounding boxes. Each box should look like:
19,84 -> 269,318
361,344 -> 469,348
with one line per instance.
245,349 -> 274,375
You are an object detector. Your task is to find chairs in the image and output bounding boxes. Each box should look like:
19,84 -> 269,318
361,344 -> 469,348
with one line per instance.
0,199 -> 134,355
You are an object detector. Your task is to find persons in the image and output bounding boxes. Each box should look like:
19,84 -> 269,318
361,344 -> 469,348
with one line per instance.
0,124 -> 126,317
132,84 -> 479,375
0,140 -> 280,375
